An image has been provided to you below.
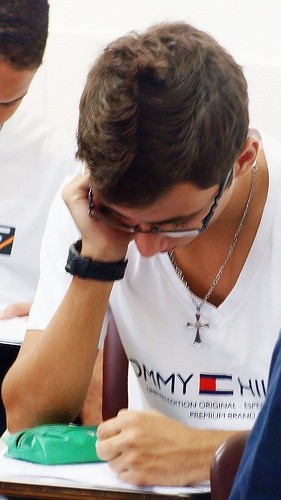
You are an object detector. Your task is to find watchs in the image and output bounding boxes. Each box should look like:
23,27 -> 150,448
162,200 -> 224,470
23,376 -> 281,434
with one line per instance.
65,239 -> 128,281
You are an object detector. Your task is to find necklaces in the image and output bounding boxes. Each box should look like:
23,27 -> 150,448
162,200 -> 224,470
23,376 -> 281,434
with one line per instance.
168,165 -> 257,348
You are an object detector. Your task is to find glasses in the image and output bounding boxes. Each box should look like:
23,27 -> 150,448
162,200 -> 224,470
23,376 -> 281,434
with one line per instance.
87,164 -> 233,240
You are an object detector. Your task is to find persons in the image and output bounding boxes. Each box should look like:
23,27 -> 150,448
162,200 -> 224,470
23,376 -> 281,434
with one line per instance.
2,17 -> 280,487
1,0 -> 87,435
